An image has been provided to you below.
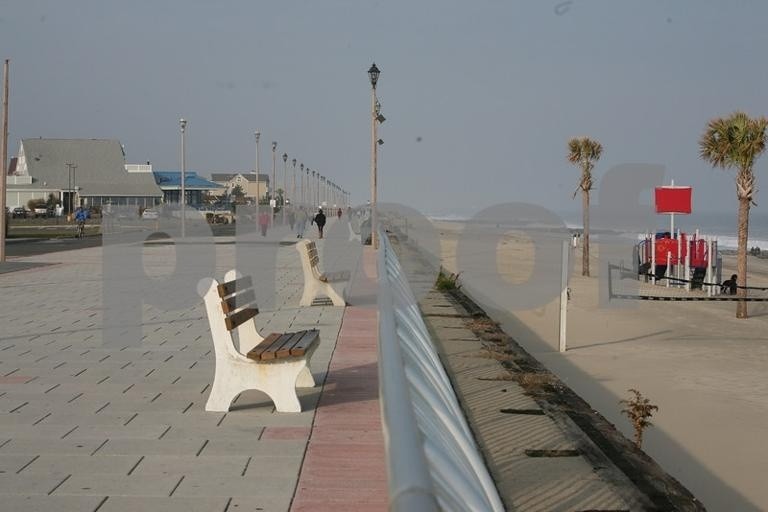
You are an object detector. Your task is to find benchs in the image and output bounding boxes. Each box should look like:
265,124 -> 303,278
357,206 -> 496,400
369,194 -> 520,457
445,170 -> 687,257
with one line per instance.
197,239 -> 351,413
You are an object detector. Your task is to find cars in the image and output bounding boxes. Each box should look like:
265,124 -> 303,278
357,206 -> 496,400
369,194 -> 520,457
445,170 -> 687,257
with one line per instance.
12,207 -> 26,218
142,208 -> 159,222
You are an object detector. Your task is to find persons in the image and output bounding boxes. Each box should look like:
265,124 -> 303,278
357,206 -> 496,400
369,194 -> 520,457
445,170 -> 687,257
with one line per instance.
259,210 -> 269,238
314,210 -> 326,238
294,206 -> 306,238
338,207 -> 342,218
73,206 -> 89,230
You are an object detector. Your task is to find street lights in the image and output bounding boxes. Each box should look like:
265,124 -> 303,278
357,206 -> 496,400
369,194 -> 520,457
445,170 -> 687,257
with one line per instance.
180,118 -> 187,240
300,162 -> 351,220
282,152 -> 288,223
367,62 -> 381,249
254,131 -> 260,237
292,158 -> 296,223
270,140 -> 277,225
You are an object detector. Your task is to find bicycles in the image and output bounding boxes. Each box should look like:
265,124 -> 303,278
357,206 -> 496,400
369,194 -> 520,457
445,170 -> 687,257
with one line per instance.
76,221 -> 84,241
